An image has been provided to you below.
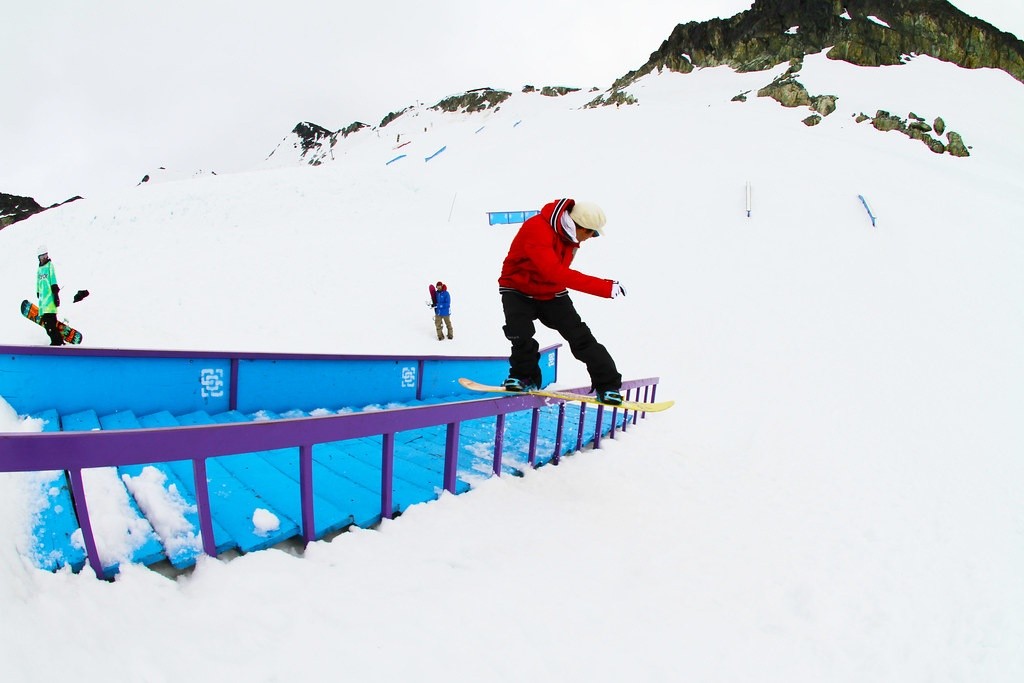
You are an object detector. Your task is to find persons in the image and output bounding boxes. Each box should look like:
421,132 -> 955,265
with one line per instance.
37,246 -> 66,346
497,198 -> 628,405
427,282 -> 453,341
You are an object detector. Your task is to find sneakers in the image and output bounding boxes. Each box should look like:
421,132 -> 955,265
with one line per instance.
595,386 -> 624,405
504,375 -> 540,392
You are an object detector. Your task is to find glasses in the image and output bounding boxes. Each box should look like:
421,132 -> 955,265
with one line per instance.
592,230 -> 599,237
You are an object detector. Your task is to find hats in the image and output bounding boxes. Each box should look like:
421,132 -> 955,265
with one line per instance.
437,282 -> 443,286
570,202 -> 608,236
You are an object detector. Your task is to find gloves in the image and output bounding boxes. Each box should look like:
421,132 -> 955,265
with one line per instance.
610,280 -> 627,300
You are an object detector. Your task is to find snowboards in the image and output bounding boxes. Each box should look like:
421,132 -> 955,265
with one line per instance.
429,284 -> 437,314
457,377 -> 675,413
20,299 -> 82,345
